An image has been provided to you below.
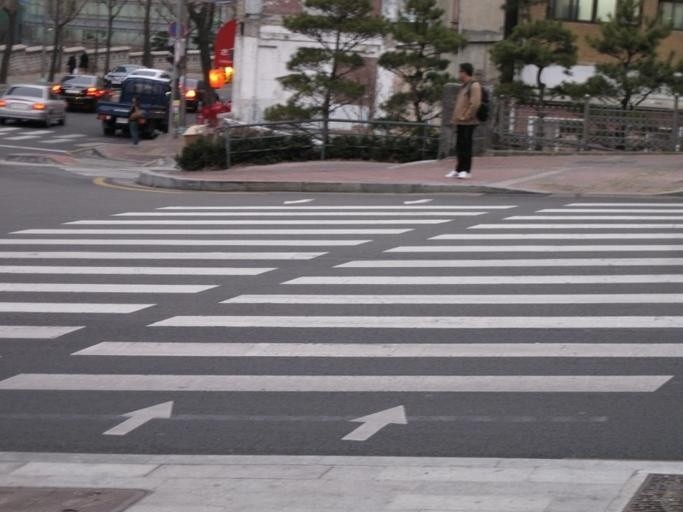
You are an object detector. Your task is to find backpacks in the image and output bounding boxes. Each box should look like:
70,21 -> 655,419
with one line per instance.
467,81 -> 490,121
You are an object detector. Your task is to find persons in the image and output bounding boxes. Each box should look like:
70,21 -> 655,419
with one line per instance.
125,95 -> 139,148
65,54 -> 76,75
444,62 -> 483,179
78,50 -> 88,76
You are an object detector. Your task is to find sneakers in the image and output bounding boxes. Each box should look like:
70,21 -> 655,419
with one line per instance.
446,171 -> 471,178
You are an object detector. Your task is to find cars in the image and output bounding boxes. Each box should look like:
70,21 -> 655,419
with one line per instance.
0,64 -> 219,128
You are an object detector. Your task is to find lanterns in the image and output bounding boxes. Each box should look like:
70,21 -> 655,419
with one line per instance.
222,66 -> 233,84
207,68 -> 223,89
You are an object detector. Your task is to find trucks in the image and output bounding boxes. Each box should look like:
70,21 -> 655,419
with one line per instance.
96,77 -> 172,136
